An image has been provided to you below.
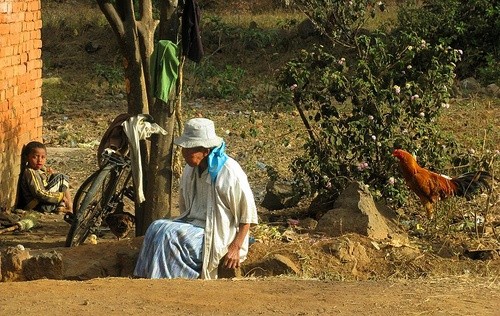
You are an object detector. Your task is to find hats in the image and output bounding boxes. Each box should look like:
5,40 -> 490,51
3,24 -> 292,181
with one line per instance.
172,118 -> 223,148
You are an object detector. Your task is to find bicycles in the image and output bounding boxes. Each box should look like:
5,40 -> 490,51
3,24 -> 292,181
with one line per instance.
63,115 -> 154,248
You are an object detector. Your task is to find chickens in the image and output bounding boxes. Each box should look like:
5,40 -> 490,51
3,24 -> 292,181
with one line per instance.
391,148 -> 492,224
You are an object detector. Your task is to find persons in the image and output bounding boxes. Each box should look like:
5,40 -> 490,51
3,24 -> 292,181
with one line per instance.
15,141 -> 75,213
133,118 -> 259,279
